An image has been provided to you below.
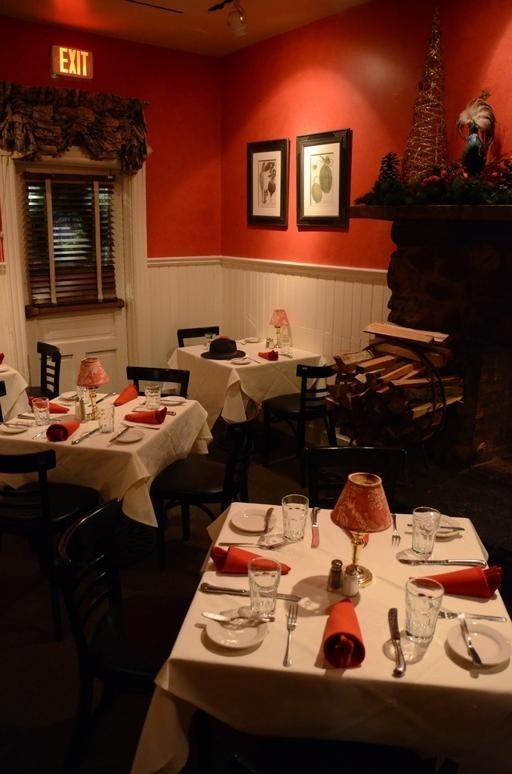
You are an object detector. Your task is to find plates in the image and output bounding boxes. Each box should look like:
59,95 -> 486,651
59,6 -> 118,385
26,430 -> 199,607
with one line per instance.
1,364 -> 9,371
231,357 -> 251,364
1,384 -> 187,445
204,606 -> 268,650
244,337 -> 260,342
231,508 -> 278,532
433,514 -> 460,537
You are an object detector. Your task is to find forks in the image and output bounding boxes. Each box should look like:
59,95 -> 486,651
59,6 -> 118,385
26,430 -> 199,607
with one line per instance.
283,603 -> 300,667
390,514 -> 400,540
218,538 -> 286,548
446,621 -> 511,666
203,613 -> 275,623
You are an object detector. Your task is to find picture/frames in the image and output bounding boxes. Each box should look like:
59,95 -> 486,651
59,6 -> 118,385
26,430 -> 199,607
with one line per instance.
247,138 -> 288,226
296,129 -> 353,227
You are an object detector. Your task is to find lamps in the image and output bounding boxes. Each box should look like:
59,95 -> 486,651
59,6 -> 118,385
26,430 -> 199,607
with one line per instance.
207,0 -> 251,39
330,471 -> 393,595
269,309 -> 289,346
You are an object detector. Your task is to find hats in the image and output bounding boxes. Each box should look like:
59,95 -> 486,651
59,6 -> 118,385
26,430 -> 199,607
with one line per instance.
200,337 -> 246,362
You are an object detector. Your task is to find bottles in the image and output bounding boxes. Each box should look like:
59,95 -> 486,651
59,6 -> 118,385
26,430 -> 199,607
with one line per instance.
343,564 -> 359,597
265,336 -> 275,348
327,558 -> 343,592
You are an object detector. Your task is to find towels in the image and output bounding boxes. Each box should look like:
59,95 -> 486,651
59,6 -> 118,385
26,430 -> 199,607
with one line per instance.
325,600 -> 365,665
409,563 -> 503,601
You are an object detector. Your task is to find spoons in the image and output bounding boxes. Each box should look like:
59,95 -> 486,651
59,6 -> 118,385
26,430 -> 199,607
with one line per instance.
438,608 -> 506,621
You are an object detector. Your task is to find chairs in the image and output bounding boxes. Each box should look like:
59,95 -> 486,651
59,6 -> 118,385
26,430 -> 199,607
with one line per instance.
170,325 -> 340,468
61,494 -> 183,751
1,340 -> 246,568
307,445 -> 408,509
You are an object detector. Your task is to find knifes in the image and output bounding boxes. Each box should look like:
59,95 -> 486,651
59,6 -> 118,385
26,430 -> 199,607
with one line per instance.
399,558 -> 486,567
311,506 -> 321,546
457,612 -> 482,665
387,607 -> 407,677
279,353 -> 293,358
406,522 -> 465,530
200,583 -> 300,603
264,507 -> 274,532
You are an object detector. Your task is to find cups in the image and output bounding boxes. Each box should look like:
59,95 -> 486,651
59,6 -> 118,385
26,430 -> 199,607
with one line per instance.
382,632 -> 426,664
405,575 -> 445,643
280,337 -> 290,354
206,335 -> 214,351
411,506 -> 441,555
247,556 -> 281,617
282,493 -> 309,541
